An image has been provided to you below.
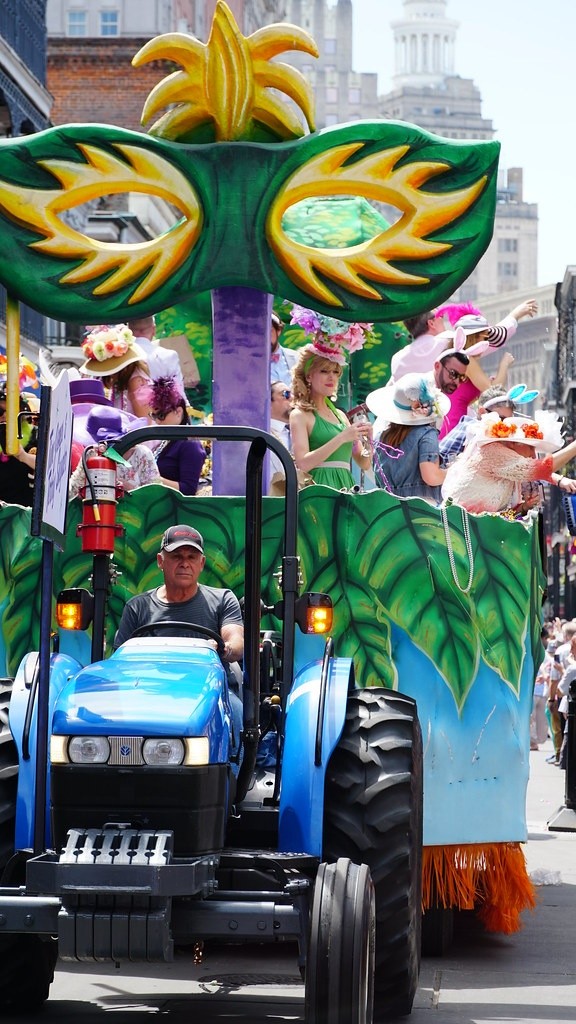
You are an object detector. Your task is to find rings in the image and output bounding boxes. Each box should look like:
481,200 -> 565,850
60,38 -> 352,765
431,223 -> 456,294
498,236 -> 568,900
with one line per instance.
531,307 -> 532,309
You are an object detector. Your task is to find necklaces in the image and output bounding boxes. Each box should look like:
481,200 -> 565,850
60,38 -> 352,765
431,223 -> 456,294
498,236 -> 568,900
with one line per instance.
321,411 -> 331,417
152,440 -> 164,457
368,436 -> 403,493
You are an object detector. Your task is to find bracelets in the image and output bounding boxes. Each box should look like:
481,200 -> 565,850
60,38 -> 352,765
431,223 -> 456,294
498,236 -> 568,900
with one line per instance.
557,476 -> 563,487
522,506 -> 525,512
548,699 -> 555,702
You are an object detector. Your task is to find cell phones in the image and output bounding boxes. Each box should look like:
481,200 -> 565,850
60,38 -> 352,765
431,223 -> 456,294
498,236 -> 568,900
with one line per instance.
554,653 -> 560,664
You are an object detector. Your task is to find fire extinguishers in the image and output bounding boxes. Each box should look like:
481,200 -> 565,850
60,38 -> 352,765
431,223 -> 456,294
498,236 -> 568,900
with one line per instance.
82,439 -> 123,554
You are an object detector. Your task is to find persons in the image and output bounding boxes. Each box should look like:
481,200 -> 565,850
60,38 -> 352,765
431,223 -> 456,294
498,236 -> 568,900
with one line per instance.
0,382 -> 37,505
365,297 -> 576,514
71,315 -> 205,493
115,525 -> 243,778
529,616 -> 576,773
271,307 -> 372,495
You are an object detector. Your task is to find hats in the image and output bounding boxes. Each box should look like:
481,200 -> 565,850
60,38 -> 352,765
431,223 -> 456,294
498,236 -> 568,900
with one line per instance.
0,393 -> 33,424
78,321 -> 143,377
69,402 -> 147,450
69,379 -> 114,406
464,409 -> 567,453
434,302 -> 492,338
289,304 -> 373,366
161,524 -> 205,553
271,309 -> 285,331
365,373 -> 450,425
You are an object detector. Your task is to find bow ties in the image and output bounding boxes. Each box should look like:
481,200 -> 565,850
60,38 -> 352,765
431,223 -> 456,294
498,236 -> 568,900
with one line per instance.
270,354 -> 279,363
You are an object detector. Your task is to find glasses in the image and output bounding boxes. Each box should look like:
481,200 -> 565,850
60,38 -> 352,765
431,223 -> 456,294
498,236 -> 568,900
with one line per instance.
428,313 -> 444,322
148,407 -> 178,421
477,333 -> 489,342
441,363 -> 467,384
272,390 -> 292,399
0,405 -> 6,416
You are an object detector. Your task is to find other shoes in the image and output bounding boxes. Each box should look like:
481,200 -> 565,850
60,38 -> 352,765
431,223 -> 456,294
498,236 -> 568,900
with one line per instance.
529,741 -> 566,771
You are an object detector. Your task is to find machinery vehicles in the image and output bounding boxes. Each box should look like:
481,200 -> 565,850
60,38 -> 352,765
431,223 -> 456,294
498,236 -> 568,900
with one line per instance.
0,425 -> 423,1015
